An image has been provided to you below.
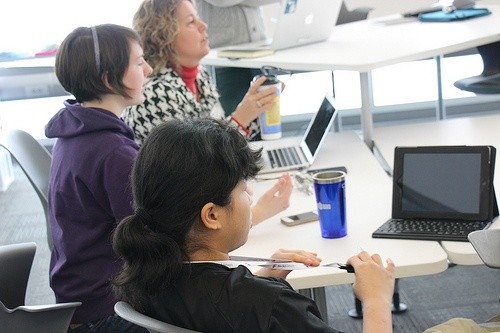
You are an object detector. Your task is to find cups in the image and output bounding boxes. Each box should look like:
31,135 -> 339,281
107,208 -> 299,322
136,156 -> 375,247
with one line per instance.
249,75 -> 282,140
312,170 -> 348,238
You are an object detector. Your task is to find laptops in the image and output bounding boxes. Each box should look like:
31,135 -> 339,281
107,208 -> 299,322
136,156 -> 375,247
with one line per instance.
371,145 -> 496,241
226,0 -> 344,53
254,93 -> 338,176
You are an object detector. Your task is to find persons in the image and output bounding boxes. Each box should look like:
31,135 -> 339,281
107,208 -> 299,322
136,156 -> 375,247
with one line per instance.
45,25 -> 295,333
111,119 -> 396,333
117,0 -> 278,152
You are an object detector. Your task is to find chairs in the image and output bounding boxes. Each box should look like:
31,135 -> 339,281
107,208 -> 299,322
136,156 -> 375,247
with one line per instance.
0,129 -> 53,254
0,243 -> 83,333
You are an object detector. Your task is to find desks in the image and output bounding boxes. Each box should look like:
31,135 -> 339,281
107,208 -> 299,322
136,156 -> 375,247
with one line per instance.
200,0 -> 500,148
372,114 -> 500,313
228,128 -> 447,330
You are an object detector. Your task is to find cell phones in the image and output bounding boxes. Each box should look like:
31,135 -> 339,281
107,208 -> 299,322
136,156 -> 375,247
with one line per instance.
281,210 -> 318,226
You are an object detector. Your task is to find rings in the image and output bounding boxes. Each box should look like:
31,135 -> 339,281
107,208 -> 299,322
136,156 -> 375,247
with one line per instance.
256,100 -> 263,108
